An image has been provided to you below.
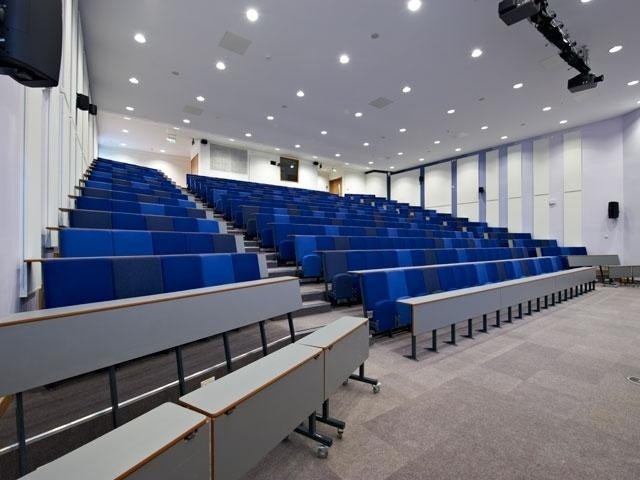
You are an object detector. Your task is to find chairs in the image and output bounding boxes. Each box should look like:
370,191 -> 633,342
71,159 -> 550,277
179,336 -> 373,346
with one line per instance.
23,158 -> 268,309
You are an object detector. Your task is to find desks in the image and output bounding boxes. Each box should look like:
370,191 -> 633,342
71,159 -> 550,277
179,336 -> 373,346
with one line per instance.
19,402 -> 212,479
178,343 -> 334,480
295,316 -> 383,436
567,254 -> 620,266
607,264 -> 639,285
396,266 -> 597,360
1,276 -> 303,400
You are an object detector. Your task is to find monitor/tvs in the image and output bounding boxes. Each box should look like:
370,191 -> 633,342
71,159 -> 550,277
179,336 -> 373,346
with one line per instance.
279,156 -> 299,182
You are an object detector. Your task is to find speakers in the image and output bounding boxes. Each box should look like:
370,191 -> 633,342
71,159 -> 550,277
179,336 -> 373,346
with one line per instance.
1,1 -> 65,89
313,161 -> 319,165
76,92 -> 91,111
607,201 -> 620,219
200,139 -> 208,145
89,104 -> 98,115
419,176 -> 424,184
478,186 -> 484,193
